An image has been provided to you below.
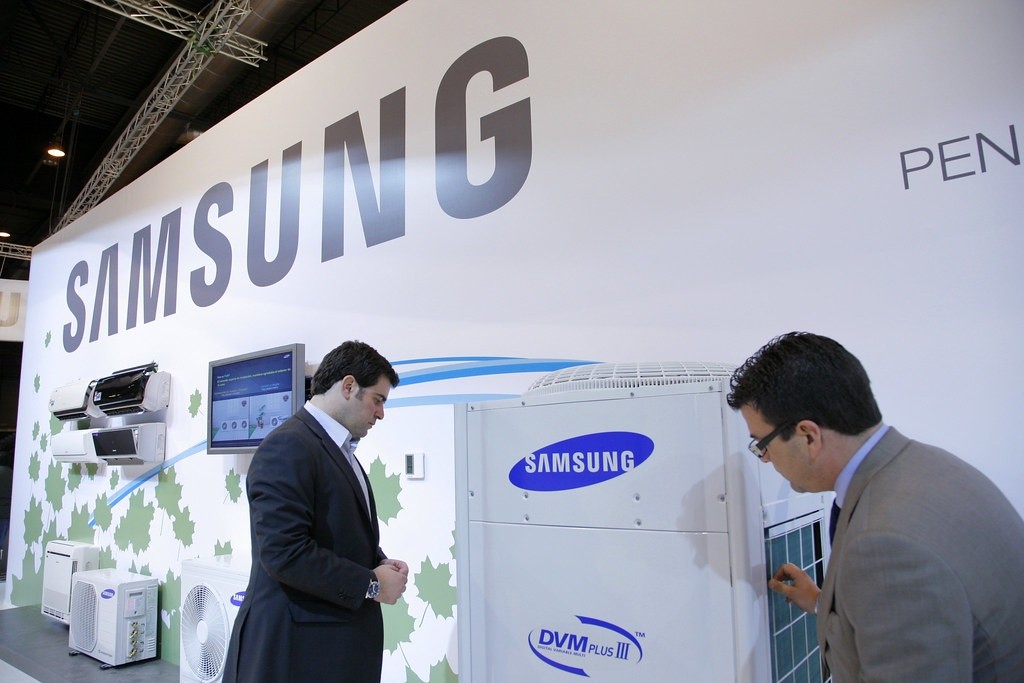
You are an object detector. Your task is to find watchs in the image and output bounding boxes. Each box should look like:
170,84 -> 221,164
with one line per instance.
366,569 -> 381,601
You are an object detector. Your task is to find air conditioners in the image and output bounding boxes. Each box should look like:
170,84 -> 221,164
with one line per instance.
39,540 -> 98,624
67,568 -> 159,669
46,360 -> 171,464
450,360 -> 831,682
178,559 -> 253,683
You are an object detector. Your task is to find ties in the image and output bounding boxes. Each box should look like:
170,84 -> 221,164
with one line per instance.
827,499 -> 839,543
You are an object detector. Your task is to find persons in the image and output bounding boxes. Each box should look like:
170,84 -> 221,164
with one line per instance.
725,329 -> 1024,682
219,338 -> 409,683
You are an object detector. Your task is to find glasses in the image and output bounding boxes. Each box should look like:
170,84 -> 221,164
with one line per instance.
748,414 -> 819,459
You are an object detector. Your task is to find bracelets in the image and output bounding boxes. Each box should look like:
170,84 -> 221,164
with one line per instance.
813,591 -> 821,617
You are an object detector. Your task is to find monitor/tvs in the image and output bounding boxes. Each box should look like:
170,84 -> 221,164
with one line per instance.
207,343 -> 305,454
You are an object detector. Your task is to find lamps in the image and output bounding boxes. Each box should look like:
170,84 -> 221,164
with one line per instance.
46,132 -> 65,156
